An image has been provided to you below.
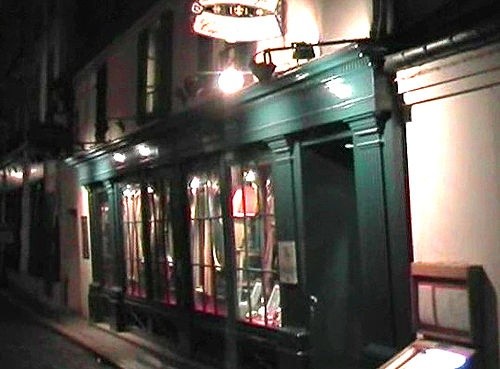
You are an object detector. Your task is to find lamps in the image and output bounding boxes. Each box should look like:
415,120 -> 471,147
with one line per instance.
248,60 -> 277,81
292,42 -> 316,61
